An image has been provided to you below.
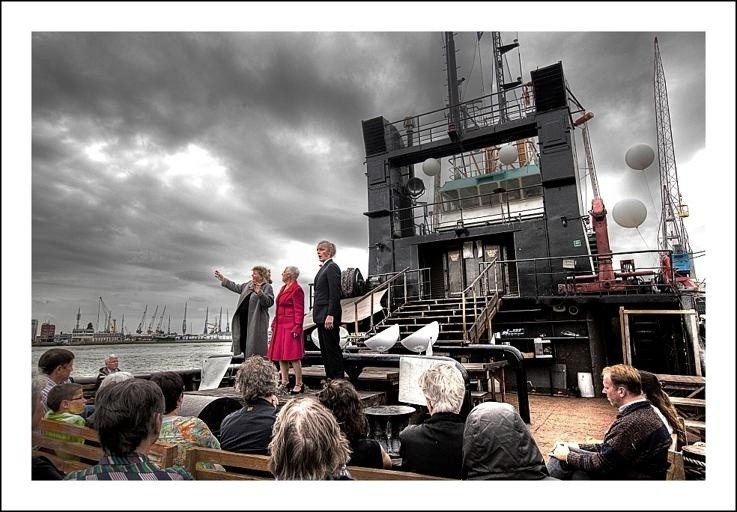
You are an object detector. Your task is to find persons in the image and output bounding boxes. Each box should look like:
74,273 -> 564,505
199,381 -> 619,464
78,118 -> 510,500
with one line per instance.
399,362 -> 550,480
546,364 -> 683,479
220,355 -> 392,480
267,266 -> 305,395
214,265 -> 274,360
312,241 -> 345,379
31,349 -> 225,480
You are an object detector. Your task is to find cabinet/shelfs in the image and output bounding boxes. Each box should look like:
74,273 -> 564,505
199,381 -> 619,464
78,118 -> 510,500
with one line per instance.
478,318 -> 587,343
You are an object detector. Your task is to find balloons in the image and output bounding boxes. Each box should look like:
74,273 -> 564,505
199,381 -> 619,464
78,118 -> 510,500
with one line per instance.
423,157 -> 441,177
612,198 -> 646,228
626,144 -> 654,170
498,146 -> 518,164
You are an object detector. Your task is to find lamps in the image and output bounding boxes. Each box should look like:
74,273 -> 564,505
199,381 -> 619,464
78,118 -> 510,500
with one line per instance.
310,325 -> 350,352
399,319 -> 440,356
405,175 -> 425,205
361,321 -> 398,353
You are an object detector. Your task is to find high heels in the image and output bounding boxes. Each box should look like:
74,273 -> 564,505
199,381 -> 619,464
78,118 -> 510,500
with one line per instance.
289,383 -> 304,395
279,383 -> 290,392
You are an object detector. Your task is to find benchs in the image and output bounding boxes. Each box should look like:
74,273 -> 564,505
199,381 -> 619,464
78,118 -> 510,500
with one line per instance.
30,358 -> 706,481
